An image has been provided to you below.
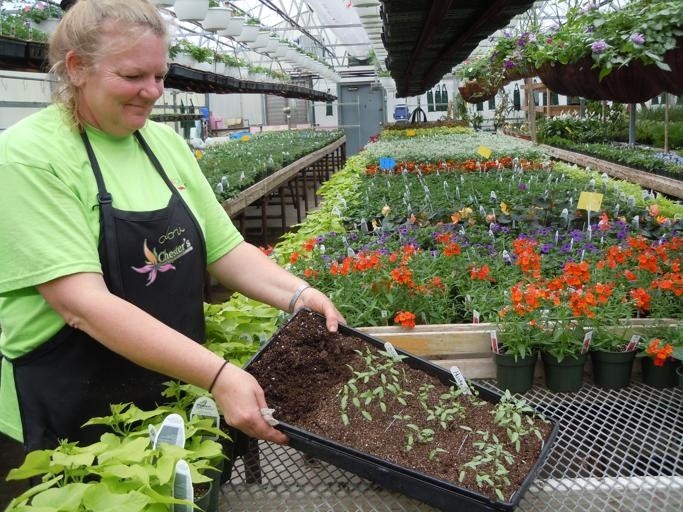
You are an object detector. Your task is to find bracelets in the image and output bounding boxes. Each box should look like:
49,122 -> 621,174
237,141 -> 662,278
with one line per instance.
206,351 -> 231,394
286,279 -> 312,314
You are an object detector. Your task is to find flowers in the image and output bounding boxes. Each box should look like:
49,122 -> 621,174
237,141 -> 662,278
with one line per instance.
450,1 -> 683,74
494,271 -> 681,368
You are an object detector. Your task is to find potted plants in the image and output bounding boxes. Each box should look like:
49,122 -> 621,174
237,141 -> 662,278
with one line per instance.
23,4 -> 66,44
165,0 -> 343,87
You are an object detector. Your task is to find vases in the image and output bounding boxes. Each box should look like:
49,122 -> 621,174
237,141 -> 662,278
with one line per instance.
497,337 -> 680,393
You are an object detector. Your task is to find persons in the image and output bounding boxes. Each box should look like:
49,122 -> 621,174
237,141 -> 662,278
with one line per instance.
0,0 -> 356,512
